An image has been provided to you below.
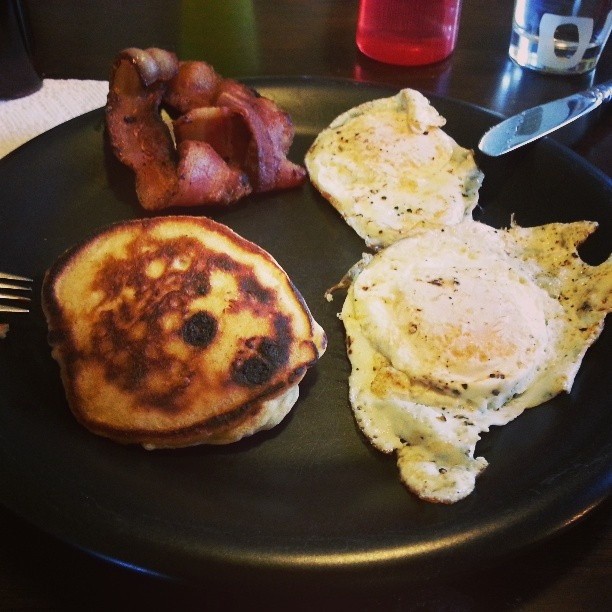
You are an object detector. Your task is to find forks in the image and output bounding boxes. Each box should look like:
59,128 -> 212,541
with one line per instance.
0,272 -> 34,313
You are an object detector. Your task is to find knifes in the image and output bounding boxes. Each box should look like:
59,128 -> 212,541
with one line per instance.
478,79 -> 612,157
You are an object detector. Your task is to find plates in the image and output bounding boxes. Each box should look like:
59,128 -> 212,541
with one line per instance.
0,76 -> 612,591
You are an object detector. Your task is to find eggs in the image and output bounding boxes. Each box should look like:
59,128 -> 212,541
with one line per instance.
304,87 -> 484,249
324,213 -> 612,506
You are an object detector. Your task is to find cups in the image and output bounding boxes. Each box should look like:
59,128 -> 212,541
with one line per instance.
508,0 -> 612,76
356,0 -> 461,66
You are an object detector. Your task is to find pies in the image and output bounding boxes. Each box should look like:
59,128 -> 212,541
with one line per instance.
39,215 -> 329,450
